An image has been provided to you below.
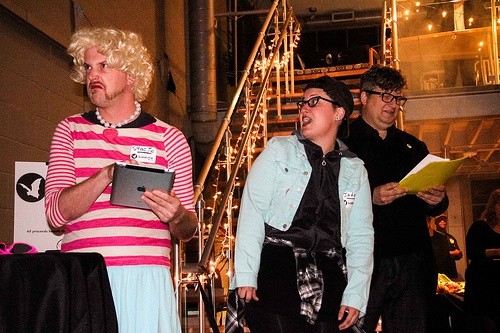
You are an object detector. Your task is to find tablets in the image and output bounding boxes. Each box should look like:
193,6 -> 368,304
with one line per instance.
110,162 -> 176,209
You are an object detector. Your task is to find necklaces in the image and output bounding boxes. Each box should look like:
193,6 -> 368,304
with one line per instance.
94,101 -> 142,129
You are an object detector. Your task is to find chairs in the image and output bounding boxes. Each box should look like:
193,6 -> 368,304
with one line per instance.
474,57 -> 500,86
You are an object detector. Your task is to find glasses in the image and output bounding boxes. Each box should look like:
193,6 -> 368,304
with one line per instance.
363,90 -> 408,106
297,95 -> 342,109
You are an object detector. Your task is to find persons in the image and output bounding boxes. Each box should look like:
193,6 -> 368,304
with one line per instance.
227,74 -> 375,333
435,214 -> 463,278
427,216 -> 449,275
45,27 -> 199,333
343,65 -> 449,333
457,189 -> 500,333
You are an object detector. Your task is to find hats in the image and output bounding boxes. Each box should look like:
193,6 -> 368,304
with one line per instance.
303,74 -> 354,138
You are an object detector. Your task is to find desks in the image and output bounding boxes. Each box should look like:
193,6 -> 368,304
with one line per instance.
0,252 -> 119,333
